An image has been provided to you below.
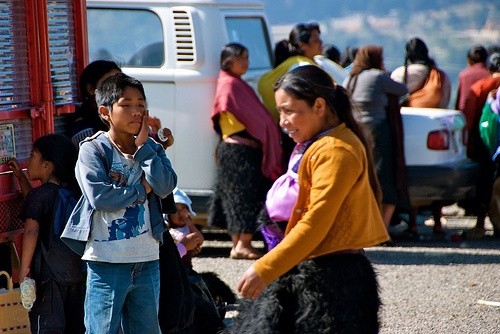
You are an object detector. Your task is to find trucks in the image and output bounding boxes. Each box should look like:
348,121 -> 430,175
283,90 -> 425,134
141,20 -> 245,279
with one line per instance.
86,0 -> 476,237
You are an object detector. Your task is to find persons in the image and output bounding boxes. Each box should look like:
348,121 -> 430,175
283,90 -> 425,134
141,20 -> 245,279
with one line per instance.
210,43 -> 282,260
8,133 -> 84,334
275,22 -> 500,240
65,58 -> 228,334
58,71 -> 179,334
238,64 -> 390,334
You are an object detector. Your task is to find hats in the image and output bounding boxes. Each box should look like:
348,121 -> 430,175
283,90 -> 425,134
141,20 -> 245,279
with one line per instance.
172,188 -> 197,218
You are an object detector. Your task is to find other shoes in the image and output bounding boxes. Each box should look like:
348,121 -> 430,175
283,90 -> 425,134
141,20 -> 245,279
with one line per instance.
230,247 -> 265,260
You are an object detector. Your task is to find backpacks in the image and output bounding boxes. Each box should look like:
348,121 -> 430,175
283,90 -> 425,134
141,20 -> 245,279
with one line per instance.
34,185 -> 86,287
